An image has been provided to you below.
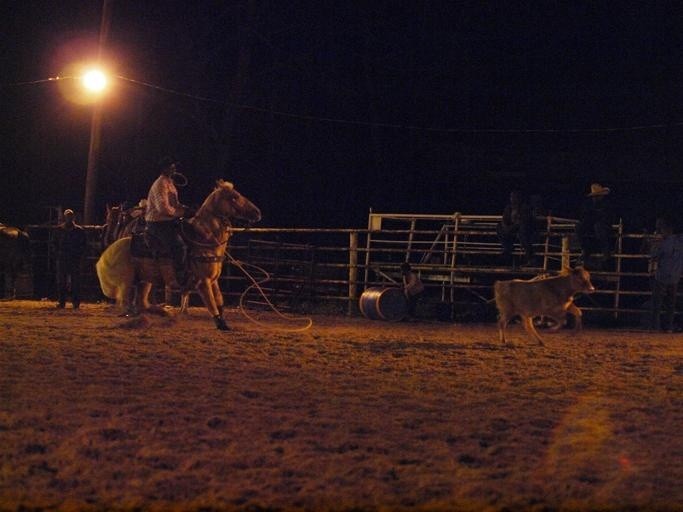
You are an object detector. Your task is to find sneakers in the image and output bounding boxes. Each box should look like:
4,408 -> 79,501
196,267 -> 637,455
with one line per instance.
72,308 -> 80,314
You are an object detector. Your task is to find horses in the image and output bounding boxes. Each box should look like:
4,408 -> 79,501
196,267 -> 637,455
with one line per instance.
0,224 -> 30,300
96,178 -> 261,330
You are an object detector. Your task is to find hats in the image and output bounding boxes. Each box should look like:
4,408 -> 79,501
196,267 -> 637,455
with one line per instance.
63,209 -> 73,216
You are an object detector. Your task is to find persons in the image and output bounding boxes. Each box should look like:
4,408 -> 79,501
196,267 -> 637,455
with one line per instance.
502,183 -> 683,333
53,209 -> 86,309
145,159 -> 196,285
401,263 -> 424,321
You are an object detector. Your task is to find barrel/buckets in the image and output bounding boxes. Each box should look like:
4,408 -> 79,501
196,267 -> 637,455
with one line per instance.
359,286 -> 409,320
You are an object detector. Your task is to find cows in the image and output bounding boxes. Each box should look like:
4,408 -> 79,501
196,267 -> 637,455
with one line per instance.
493,260 -> 594,345
